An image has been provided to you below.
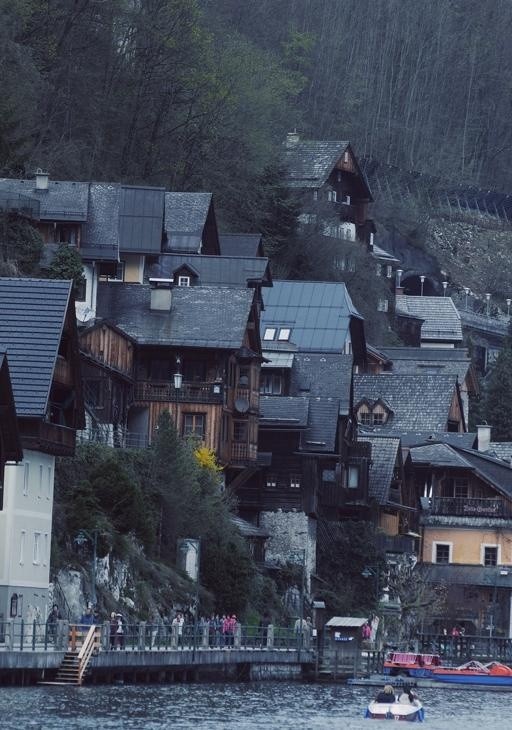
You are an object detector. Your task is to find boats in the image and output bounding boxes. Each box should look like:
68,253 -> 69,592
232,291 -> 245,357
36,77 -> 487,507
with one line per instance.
347,652 -> 512,691
363,695 -> 424,722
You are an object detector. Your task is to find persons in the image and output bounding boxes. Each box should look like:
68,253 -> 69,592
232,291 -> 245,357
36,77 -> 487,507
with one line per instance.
397,685 -> 418,705
374,685 -> 396,703
437,624 -> 466,657
44,604 -> 313,652
361,621 -> 372,640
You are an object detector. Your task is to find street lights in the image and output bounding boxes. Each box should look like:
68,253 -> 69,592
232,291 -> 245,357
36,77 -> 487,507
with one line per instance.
289,548 -> 306,647
480,572 -> 497,620
73,529 -> 98,610
396,269 -> 511,318
361,560 -> 379,612
179,536 -> 203,625
173,357 -> 183,440
